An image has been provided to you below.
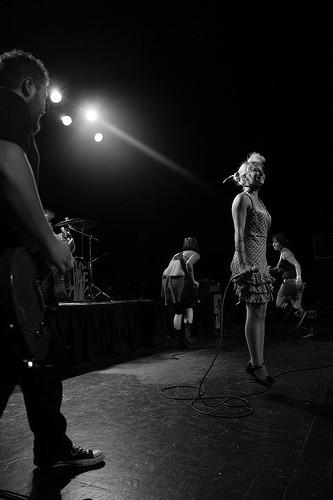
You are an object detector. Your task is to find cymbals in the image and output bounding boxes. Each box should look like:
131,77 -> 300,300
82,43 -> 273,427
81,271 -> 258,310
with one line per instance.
43,209 -> 55,220
55,218 -> 79,226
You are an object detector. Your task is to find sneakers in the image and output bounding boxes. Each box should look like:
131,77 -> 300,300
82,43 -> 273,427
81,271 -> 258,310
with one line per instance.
39,447 -> 105,469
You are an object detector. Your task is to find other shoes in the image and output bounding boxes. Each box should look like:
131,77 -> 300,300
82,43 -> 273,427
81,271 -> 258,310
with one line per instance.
247,360 -> 273,387
302,330 -> 313,339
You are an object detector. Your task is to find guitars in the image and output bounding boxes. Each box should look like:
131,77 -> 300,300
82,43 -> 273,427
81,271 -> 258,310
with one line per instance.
2,231 -> 76,346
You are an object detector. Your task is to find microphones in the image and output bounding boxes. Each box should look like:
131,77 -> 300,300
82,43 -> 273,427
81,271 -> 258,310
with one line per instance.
232,265 -> 260,280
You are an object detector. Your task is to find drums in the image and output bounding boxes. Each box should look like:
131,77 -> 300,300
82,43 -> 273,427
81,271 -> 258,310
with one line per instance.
50,256 -> 93,302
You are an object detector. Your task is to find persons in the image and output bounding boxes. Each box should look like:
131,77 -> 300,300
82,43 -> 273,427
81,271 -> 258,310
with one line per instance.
268,233 -> 313,338
0,50 -> 106,468
223,152 -> 275,387
161,237 -> 200,349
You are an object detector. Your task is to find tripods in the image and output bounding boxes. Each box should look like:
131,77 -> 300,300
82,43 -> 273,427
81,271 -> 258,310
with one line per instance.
67,224 -> 113,302
